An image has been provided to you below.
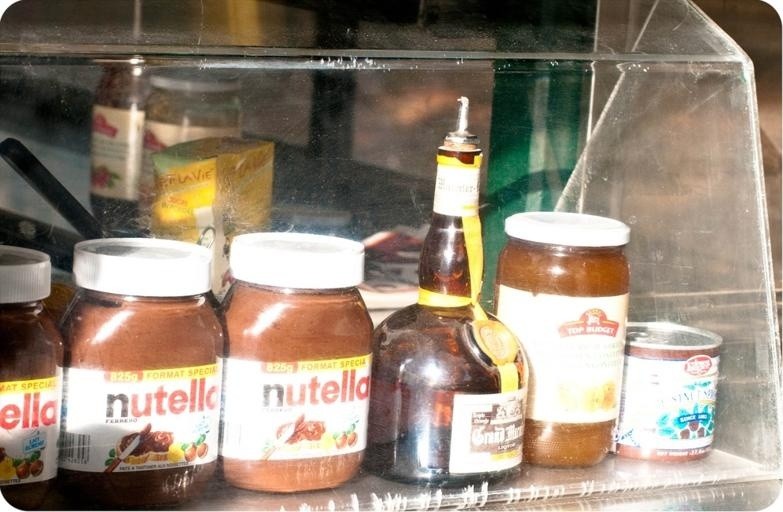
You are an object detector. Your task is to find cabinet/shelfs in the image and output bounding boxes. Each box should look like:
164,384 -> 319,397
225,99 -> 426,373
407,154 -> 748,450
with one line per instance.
0,1 -> 782,509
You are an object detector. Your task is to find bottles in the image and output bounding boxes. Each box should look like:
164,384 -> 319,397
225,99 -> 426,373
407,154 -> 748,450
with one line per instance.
368,129 -> 534,487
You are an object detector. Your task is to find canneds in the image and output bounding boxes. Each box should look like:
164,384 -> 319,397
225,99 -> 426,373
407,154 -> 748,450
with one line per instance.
58,237 -> 224,509
608,321 -> 723,463
138,71 -> 242,231
220,232 -> 375,494
0,243 -> 65,511
494,211 -> 631,467
